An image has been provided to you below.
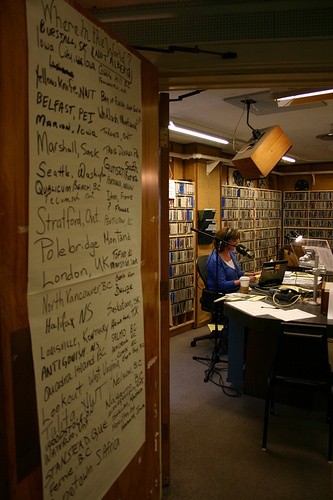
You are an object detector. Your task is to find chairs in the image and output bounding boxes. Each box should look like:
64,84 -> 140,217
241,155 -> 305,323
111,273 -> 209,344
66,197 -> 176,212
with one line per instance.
190,255 -> 228,353
262,321 -> 333,461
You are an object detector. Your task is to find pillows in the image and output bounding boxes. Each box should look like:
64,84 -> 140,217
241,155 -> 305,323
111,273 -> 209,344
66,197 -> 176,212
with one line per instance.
284,249 -> 298,266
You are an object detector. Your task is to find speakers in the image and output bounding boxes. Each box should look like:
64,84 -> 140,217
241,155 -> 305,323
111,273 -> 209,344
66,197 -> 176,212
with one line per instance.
231,125 -> 293,180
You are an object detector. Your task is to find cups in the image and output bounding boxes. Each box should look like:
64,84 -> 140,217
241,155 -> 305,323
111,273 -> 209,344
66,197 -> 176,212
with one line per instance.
239,276 -> 250,294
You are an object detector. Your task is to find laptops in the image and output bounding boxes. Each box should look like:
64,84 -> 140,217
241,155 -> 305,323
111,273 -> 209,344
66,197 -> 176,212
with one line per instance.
251,260 -> 288,288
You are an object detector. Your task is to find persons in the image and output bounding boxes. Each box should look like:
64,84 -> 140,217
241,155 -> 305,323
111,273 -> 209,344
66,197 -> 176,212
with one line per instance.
207,227 -> 261,352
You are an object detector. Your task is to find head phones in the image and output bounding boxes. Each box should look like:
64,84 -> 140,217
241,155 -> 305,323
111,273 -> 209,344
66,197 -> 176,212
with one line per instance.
219,228 -> 232,246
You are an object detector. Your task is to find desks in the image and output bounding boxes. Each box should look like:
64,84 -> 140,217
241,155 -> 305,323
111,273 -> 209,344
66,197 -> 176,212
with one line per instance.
224,269 -> 333,411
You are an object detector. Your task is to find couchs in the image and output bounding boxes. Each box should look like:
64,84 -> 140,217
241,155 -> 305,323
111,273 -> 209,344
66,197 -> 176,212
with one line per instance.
279,245 -> 333,271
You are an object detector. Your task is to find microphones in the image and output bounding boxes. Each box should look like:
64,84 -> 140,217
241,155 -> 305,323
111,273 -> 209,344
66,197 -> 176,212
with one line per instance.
236,244 -> 254,257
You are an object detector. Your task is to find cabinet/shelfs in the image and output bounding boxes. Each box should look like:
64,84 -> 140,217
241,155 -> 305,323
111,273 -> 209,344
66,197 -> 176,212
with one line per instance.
221,185 -> 282,272
283,191 -> 333,249
169,179 -> 195,331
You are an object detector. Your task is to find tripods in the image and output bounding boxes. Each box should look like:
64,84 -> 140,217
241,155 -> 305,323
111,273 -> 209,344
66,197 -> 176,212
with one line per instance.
191,227 -> 236,382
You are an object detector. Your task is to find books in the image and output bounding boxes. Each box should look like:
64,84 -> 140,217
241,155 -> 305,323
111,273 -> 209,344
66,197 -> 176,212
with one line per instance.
169,180 -> 333,329
